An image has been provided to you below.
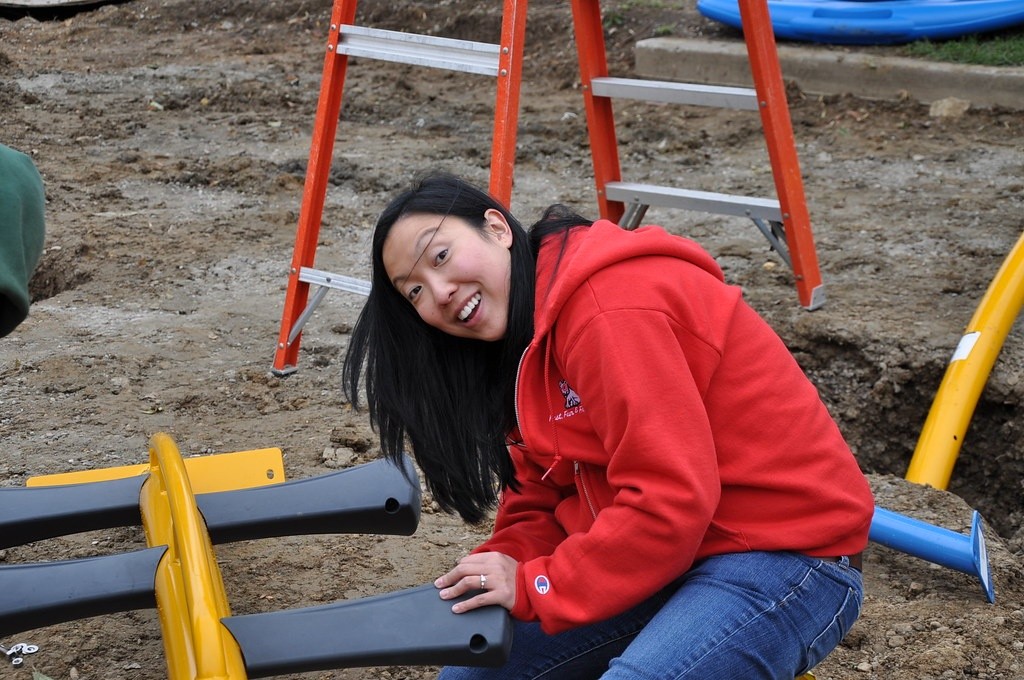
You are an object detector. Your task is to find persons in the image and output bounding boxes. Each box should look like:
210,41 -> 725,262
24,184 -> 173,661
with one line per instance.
346,172 -> 873,680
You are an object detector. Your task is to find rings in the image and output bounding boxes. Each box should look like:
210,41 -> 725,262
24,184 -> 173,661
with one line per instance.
480,575 -> 486,589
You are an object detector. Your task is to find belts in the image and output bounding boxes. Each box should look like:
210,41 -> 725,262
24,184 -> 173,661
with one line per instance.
816,554 -> 862,572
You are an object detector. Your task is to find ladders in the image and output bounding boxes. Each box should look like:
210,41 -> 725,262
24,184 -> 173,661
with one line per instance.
273,0 -> 826,379
